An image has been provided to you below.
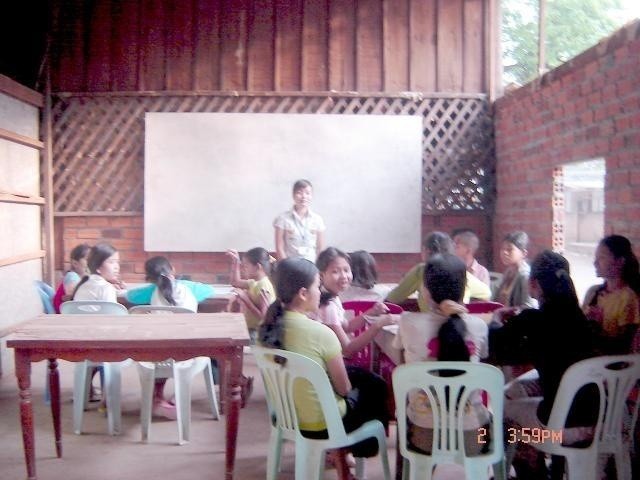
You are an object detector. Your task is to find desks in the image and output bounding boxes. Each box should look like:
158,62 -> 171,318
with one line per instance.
6,312 -> 251,461
117,283 -> 247,312
372,282 -> 420,312
365,314 -> 534,430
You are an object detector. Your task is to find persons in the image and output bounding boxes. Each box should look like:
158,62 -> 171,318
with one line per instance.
255,256 -> 389,479
341,251 -> 383,364
391,252 -> 489,456
211,247 -> 279,409
451,228 -> 492,305
384,231 -> 471,314
491,231 -> 535,313
126,255 -> 215,420
273,180 -> 327,264
503,234 -> 640,480
313,247 -> 398,469
490,249 -> 608,479
71,242 -> 133,419
53,243 -> 106,403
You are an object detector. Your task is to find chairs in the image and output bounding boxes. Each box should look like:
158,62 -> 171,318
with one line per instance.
394,357 -> 507,480
341,301 -> 403,381
127,305 -> 221,445
35,280 -> 104,406
249,345 -> 392,480
60,299 -> 134,437
463,302 -> 506,409
508,353 -> 639,479
490,271 -> 506,301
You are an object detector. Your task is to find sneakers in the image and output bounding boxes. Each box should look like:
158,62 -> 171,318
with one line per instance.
154,399 -> 177,420
240,374 -> 253,408
98,404 -> 111,414
